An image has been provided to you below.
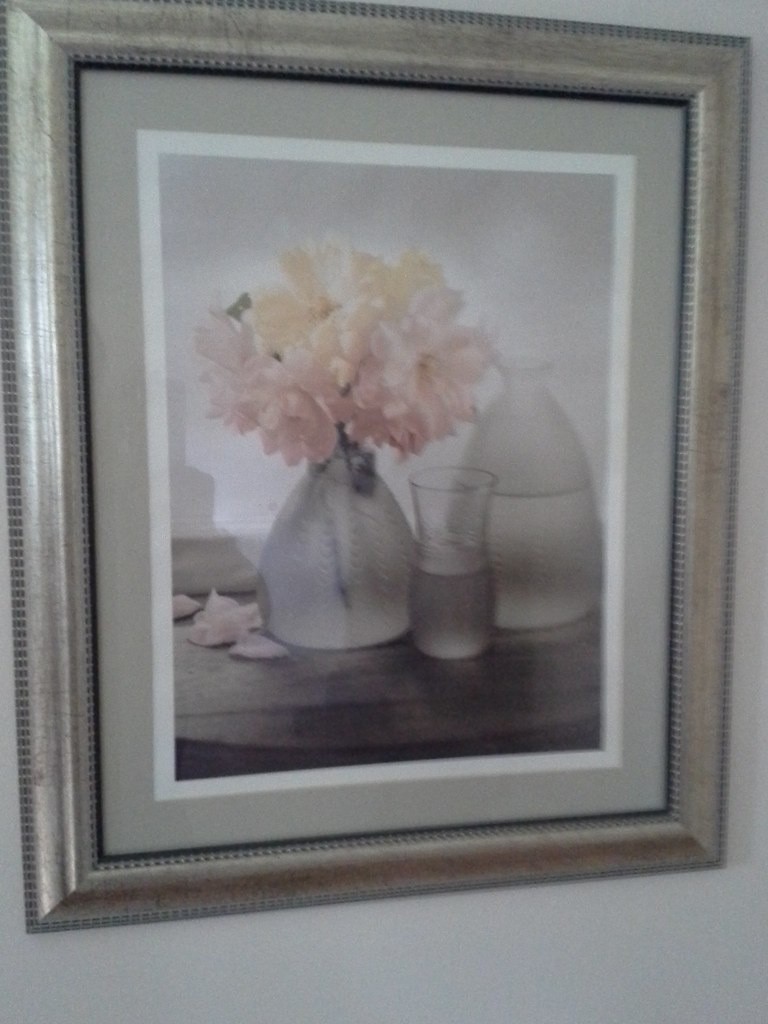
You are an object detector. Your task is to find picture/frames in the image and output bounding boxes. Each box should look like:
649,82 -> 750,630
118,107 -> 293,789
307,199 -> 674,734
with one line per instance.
0,3 -> 756,936
